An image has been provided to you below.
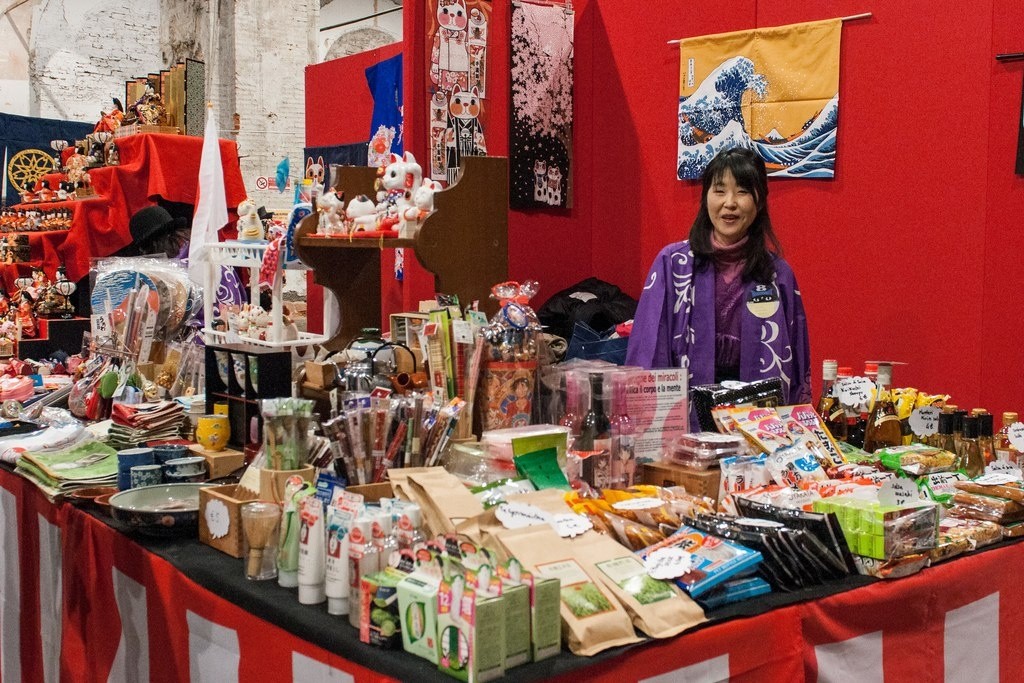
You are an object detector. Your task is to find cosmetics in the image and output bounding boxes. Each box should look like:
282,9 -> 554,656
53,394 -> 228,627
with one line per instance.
278,475 -> 561,683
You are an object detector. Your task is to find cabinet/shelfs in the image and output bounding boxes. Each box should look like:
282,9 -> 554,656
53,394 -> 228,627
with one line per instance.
205,343 -> 291,451
293,154 -> 510,353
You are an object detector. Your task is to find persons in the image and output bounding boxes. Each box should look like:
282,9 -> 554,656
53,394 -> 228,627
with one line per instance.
0,265 -> 71,337
0,77 -> 167,260
625,147 -> 812,431
129,206 -> 250,348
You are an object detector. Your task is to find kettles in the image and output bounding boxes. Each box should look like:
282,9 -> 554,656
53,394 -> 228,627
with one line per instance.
331,337 -> 417,392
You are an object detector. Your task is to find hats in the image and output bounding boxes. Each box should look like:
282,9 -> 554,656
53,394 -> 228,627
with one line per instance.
128,206 -> 172,243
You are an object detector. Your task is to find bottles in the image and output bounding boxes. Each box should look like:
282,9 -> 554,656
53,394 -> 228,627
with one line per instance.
937,405 -> 1024,478
817,359 -> 902,454
559,376 -> 635,490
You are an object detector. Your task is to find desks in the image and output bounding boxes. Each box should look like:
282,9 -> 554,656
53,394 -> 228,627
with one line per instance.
0,460 -> 1024,683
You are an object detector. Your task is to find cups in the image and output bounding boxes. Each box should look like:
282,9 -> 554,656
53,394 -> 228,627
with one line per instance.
476,361 -> 535,431
214,350 -> 258,397
196,415 -> 231,451
258,464 -> 315,503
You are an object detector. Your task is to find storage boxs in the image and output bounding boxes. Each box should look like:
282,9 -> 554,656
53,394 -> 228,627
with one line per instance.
397,550 -> 562,683
812,496 -> 939,558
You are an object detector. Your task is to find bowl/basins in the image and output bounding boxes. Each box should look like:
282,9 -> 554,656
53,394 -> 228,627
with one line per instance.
73,444 -> 223,532
